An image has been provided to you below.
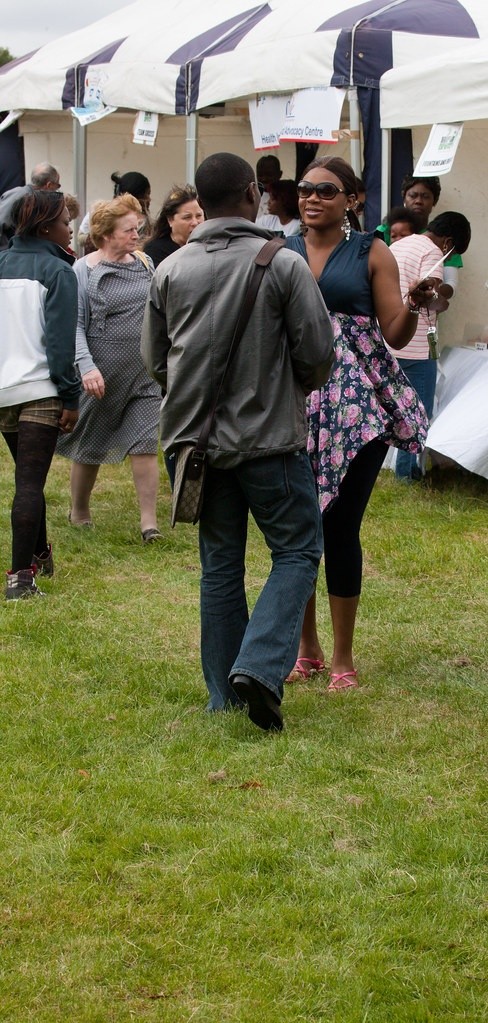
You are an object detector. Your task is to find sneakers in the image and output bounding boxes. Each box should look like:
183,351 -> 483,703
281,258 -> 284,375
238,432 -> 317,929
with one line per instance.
6,569 -> 47,602
34,543 -> 54,579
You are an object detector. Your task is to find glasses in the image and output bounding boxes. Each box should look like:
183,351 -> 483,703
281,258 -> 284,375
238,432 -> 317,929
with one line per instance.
169,191 -> 183,200
297,181 -> 348,200
50,180 -> 61,188
354,201 -> 365,212
245,182 -> 264,196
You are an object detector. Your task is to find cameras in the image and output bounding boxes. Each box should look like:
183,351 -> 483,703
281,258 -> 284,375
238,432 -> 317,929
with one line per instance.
427,327 -> 440,359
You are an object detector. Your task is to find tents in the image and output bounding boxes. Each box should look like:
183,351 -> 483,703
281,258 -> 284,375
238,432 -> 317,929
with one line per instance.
0,0 -> 488,348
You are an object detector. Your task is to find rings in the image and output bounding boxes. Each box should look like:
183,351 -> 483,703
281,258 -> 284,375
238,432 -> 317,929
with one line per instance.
432,292 -> 440,300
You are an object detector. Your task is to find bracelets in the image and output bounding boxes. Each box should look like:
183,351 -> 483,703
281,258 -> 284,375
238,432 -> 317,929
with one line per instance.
406,296 -> 419,315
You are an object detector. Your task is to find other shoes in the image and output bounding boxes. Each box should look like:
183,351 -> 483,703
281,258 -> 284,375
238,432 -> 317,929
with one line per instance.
69,514 -> 92,528
232,675 -> 284,731
141,528 -> 163,541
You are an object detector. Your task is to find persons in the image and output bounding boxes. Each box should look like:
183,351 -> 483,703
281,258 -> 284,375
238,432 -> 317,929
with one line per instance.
140,151 -> 336,732
0,189 -> 79,603
0,154 -> 474,481
68,192 -> 164,542
274,156 -> 433,695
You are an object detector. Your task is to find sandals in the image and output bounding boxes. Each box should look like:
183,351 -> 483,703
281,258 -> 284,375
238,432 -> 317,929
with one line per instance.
327,669 -> 359,692
285,658 -> 325,682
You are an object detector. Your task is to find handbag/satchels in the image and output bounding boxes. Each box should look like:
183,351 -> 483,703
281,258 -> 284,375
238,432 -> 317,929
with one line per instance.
171,446 -> 206,527
